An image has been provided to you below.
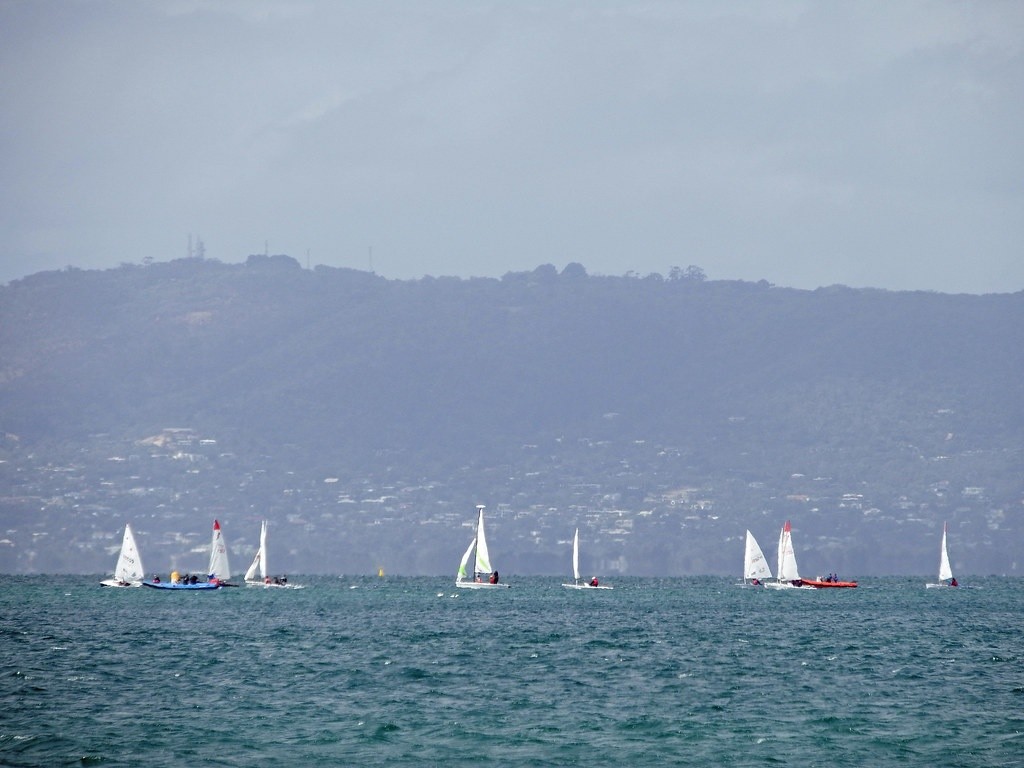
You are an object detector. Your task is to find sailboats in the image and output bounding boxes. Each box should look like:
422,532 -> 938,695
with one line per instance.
736,529 -> 772,590
925,519 -> 980,590
195,518 -> 238,587
244,518 -> 293,590
99,523 -> 144,588
762,520 -> 817,590
561,526 -> 614,590
455,505 -> 509,590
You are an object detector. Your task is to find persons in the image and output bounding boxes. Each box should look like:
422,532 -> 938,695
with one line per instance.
820,572 -> 839,584
789,578 -> 803,587
264,574 -> 288,586
475,570 -> 500,584
950,577 -> 959,587
169,567 -> 180,585
752,578 -> 762,586
182,572 -> 216,585
590,576 -> 600,587
151,575 -> 160,583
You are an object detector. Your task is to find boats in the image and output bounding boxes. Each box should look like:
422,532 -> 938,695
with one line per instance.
144,580 -> 217,590
800,576 -> 859,588
